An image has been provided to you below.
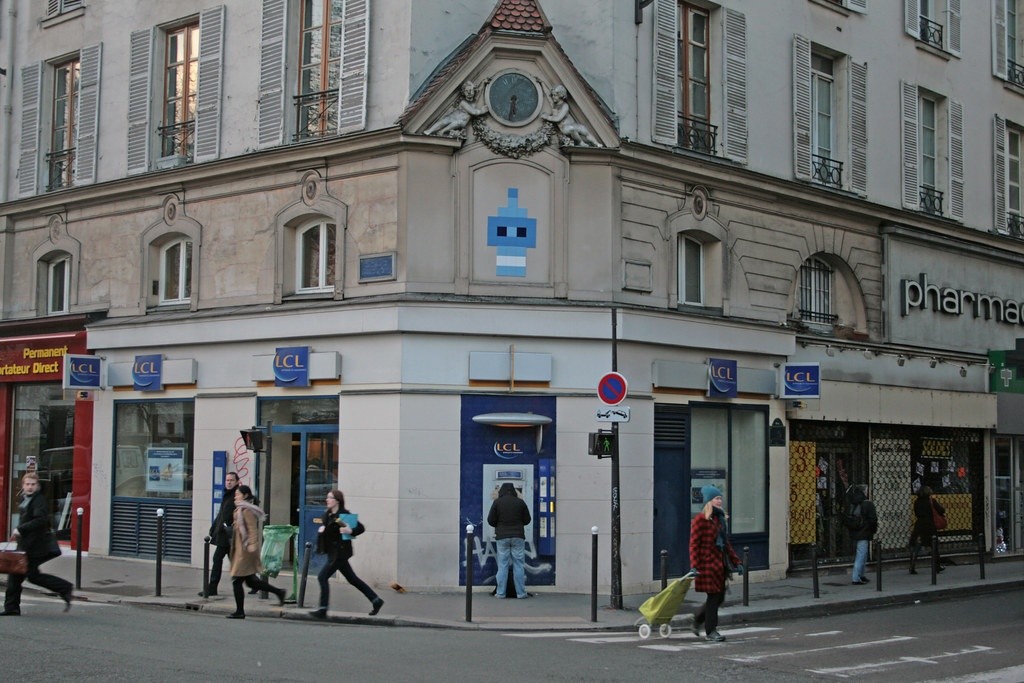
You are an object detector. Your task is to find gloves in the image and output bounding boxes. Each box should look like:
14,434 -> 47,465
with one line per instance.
690,568 -> 696,576
737,564 -> 744,575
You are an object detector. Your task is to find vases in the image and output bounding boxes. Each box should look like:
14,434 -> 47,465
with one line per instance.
156,155 -> 187,169
851,330 -> 869,342
834,323 -> 854,340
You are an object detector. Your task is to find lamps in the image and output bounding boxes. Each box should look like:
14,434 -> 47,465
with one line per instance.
968,362 -> 995,374
940,358 -> 967,378
826,345 -> 834,356
897,355 -> 905,366
864,348 -> 872,359
930,357 -> 937,368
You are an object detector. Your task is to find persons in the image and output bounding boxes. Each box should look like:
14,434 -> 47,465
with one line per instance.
908,486 -> 946,575
309,490 -> 384,617
689,485 -> 744,642
225,485 -> 287,617
486,483 -> 532,599
847,484 -> 878,586
199,471 -> 262,596
0,472 -> 73,616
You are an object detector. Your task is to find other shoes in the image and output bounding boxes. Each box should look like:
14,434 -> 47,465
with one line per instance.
860,576 -> 870,582
369,598 -> 384,615
310,609 -> 327,620
248,588 -> 259,595
937,564 -> 946,573
278,590 -> 286,607
852,581 -> 866,585
690,622 -> 700,636
0,610 -> 22,616
197,590 -> 218,596
705,629 -> 726,641
61,584 -> 73,603
909,567 -> 918,575
225,611 -> 246,619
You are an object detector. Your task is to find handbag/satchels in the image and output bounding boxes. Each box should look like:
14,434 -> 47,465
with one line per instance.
0,535 -> 28,574
930,496 -> 947,530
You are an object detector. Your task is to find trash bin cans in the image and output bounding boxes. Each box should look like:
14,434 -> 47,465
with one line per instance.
260,524 -> 293,579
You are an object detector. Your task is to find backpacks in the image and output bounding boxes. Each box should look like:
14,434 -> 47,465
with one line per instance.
842,501 -> 864,532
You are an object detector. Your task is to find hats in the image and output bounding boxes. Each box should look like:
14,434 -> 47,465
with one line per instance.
701,485 -> 722,507
